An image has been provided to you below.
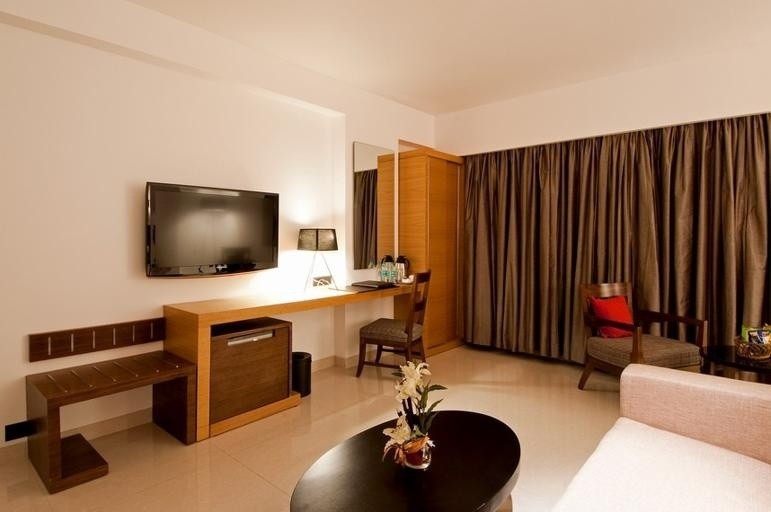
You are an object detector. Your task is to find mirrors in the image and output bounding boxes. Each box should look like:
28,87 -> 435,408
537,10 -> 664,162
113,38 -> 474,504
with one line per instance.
351,141 -> 396,271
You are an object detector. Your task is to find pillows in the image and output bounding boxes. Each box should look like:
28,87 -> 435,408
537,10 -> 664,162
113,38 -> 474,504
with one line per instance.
588,293 -> 636,339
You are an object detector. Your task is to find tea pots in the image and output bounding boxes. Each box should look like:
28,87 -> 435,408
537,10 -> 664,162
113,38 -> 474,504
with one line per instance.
395,256 -> 411,282
381,255 -> 395,264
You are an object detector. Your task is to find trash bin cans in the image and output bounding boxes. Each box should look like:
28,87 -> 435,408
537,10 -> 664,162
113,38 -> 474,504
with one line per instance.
292,352 -> 311,398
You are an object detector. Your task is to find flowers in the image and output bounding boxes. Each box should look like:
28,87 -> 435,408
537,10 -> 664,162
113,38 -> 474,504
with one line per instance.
378,360 -> 449,464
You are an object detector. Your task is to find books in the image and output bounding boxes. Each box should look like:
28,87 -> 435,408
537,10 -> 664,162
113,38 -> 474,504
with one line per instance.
353,279 -> 395,288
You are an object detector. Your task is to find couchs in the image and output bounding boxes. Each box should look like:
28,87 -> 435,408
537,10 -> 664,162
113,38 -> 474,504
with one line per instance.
545,362 -> 771,512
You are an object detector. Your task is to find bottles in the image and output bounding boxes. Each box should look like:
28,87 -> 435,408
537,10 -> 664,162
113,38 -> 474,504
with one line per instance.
368,260 -> 376,269
381,263 -> 396,283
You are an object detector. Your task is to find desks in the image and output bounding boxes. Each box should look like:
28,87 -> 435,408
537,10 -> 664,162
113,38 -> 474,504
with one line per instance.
163,281 -> 414,445
699,344 -> 770,385
23,349 -> 196,497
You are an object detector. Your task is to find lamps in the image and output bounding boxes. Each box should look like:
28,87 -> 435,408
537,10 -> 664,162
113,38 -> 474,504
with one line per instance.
296,227 -> 339,294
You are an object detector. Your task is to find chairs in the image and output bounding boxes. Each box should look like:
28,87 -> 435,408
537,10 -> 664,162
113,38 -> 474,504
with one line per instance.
575,280 -> 710,391
354,269 -> 431,379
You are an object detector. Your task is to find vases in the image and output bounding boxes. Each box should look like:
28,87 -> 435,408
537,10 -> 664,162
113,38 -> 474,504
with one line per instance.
398,446 -> 432,472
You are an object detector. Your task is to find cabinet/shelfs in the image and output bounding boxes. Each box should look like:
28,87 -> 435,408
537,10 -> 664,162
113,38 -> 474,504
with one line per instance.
376,153 -> 394,266
396,145 -> 467,360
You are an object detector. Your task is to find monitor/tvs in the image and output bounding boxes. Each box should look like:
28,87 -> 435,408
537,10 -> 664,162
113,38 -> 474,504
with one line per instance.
145,181 -> 278,276
